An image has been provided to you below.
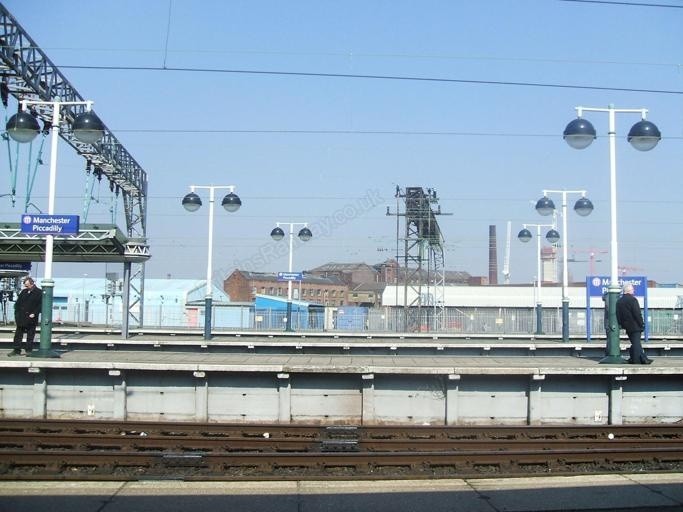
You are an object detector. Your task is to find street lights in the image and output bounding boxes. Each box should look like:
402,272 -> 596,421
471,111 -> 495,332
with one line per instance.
517,189 -> 594,343
182,184 -> 242,340
270,222 -> 313,332
6,96 -> 106,361
562,102 -> 662,365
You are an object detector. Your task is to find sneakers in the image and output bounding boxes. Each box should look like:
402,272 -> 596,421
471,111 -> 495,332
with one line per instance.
7,351 -> 32,357
629,358 -> 653,364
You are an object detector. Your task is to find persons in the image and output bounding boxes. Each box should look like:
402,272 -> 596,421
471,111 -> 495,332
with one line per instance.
7,278 -> 42,357
616,283 -> 655,365
